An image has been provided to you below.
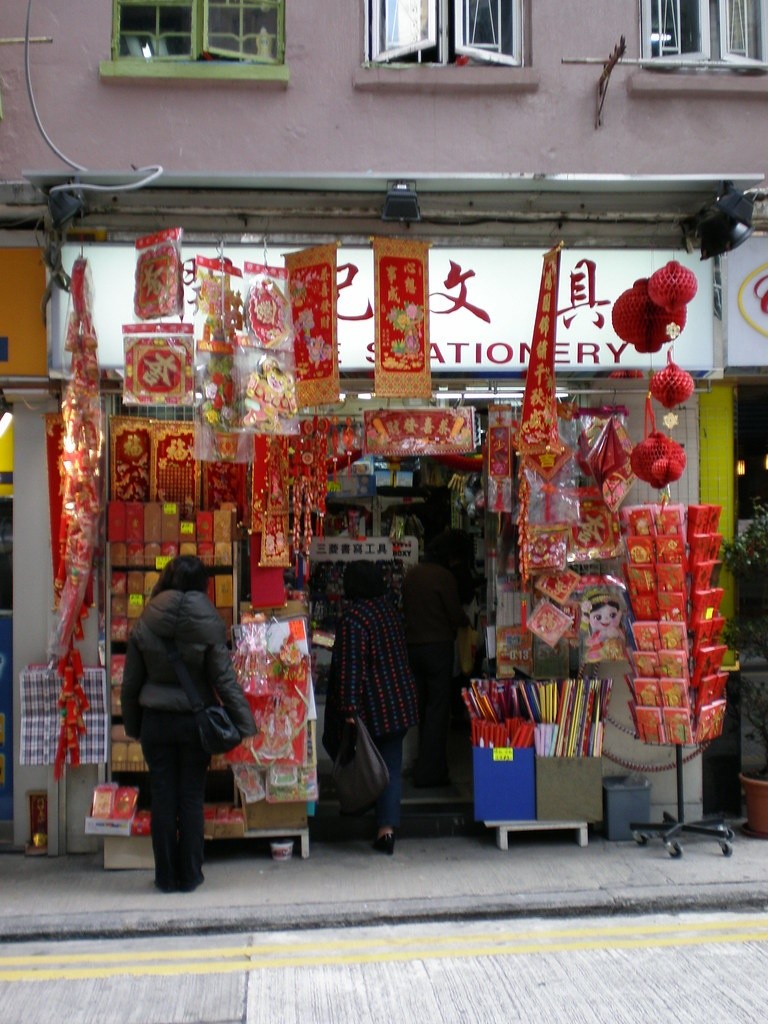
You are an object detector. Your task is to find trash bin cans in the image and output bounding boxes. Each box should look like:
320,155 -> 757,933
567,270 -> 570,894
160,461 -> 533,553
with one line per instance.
601,773 -> 652,842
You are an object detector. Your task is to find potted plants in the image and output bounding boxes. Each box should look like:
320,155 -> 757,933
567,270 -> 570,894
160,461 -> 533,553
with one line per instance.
721,602 -> 768,839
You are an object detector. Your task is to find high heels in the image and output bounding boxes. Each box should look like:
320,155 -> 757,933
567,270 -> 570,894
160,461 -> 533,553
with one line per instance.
372,833 -> 395,855
338,799 -> 376,817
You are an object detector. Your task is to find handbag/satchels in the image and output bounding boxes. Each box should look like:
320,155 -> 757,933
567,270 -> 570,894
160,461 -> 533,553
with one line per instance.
330,715 -> 390,813
16,661 -> 107,764
196,706 -> 241,754
450,625 -> 480,676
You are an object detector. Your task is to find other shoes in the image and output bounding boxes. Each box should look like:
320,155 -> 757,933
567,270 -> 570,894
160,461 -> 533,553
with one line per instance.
153,869 -> 204,892
414,772 -> 451,788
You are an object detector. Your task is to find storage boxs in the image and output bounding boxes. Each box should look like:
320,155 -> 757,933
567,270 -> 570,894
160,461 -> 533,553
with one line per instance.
246,800 -> 307,830
472,747 -> 603,822
103,837 -> 156,870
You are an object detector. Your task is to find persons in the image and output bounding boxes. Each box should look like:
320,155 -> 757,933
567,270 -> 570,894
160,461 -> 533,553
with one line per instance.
399,534 -> 471,790
324,559 -> 421,854
121,553 -> 258,893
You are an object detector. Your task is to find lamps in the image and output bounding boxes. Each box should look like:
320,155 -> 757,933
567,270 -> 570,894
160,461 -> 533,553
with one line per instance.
677,180 -> 755,261
381,179 -> 422,222
41,176 -> 88,231
